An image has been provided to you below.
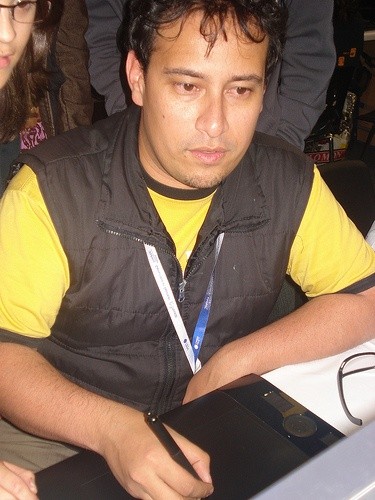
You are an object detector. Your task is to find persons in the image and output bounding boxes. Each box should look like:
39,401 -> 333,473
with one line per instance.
85,1 -> 336,154
1,0 -> 375,500
0,0 -> 37,500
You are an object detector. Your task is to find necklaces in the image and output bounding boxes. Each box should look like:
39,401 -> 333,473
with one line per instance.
144,233 -> 225,375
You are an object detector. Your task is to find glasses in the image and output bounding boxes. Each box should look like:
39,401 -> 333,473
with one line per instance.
0,0 -> 51,24
337,352 -> 375,426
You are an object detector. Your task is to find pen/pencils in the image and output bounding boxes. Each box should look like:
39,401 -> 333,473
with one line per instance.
142,405 -> 204,483
